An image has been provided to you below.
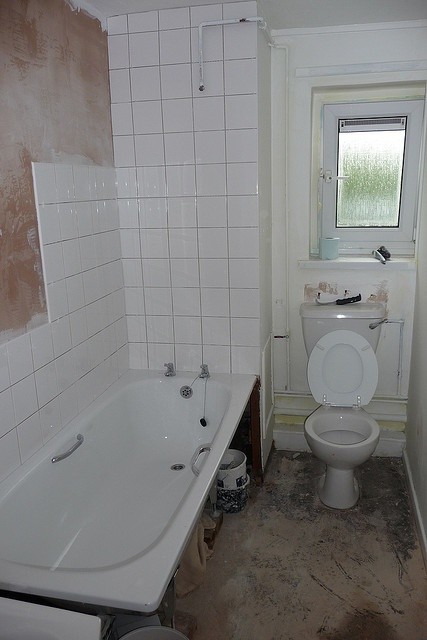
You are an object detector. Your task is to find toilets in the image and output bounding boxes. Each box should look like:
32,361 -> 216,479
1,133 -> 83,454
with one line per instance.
298,302 -> 386,511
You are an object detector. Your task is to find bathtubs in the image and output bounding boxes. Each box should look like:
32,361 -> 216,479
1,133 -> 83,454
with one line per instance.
1,369 -> 258,614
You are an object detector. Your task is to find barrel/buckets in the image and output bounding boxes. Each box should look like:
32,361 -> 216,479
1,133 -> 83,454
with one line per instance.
216,448 -> 248,489
217,473 -> 251,514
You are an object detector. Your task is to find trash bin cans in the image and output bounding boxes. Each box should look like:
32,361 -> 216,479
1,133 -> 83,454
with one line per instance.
210,448 -> 250,513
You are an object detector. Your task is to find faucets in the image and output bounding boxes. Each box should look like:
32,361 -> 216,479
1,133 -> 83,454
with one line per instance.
163,362 -> 177,377
199,363 -> 210,378
